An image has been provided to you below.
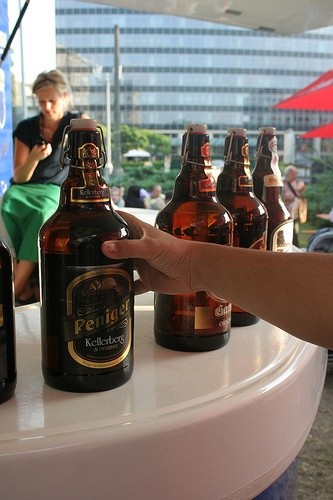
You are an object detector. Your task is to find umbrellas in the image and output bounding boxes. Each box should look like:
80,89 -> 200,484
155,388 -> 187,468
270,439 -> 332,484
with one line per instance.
274,69 -> 333,139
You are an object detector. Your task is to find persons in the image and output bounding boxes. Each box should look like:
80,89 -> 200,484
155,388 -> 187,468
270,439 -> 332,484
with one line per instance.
1,66 -> 96,307
280,167 -> 301,249
103,211 -> 333,350
111,184 -> 173,210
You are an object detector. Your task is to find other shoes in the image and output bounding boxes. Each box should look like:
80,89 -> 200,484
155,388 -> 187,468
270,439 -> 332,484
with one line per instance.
15,294 -> 37,307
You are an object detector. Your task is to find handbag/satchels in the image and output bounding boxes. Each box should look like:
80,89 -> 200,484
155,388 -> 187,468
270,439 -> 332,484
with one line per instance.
288,196 -> 308,223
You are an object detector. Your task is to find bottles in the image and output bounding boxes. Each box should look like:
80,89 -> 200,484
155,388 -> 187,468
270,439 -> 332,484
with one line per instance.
216,128 -> 269,326
0,238 -> 18,402
37,118 -> 134,393
153,123 -> 233,353
250,127 -> 294,254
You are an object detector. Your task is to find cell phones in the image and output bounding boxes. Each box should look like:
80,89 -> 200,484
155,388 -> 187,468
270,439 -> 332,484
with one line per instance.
35,140 -> 45,147
